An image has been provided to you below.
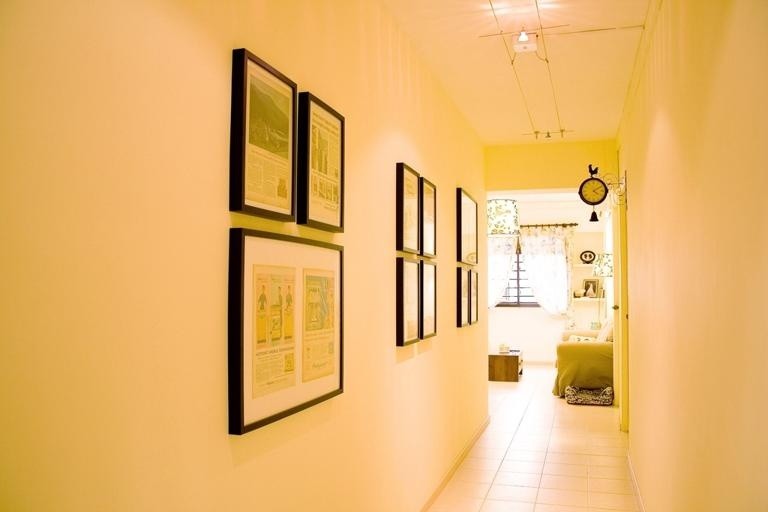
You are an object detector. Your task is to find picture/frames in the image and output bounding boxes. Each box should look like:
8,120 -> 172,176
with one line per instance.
583,279 -> 599,298
456,185 -> 479,327
394,160 -> 437,345
229,47 -> 298,224
229,227 -> 344,437
297,91 -> 344,234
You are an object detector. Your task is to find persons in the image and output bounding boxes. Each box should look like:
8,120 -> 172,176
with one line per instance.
586,282 -> 595,296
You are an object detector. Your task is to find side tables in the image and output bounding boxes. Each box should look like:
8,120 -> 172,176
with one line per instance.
488,349 -> 523,382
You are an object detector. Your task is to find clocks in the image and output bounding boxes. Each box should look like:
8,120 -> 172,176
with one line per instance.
578,164 -> 628,223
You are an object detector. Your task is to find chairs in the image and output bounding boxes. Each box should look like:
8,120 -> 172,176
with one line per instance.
552,316 -> 613,398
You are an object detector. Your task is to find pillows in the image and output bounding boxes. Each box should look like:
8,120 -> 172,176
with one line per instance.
569,334 -> 592,342
